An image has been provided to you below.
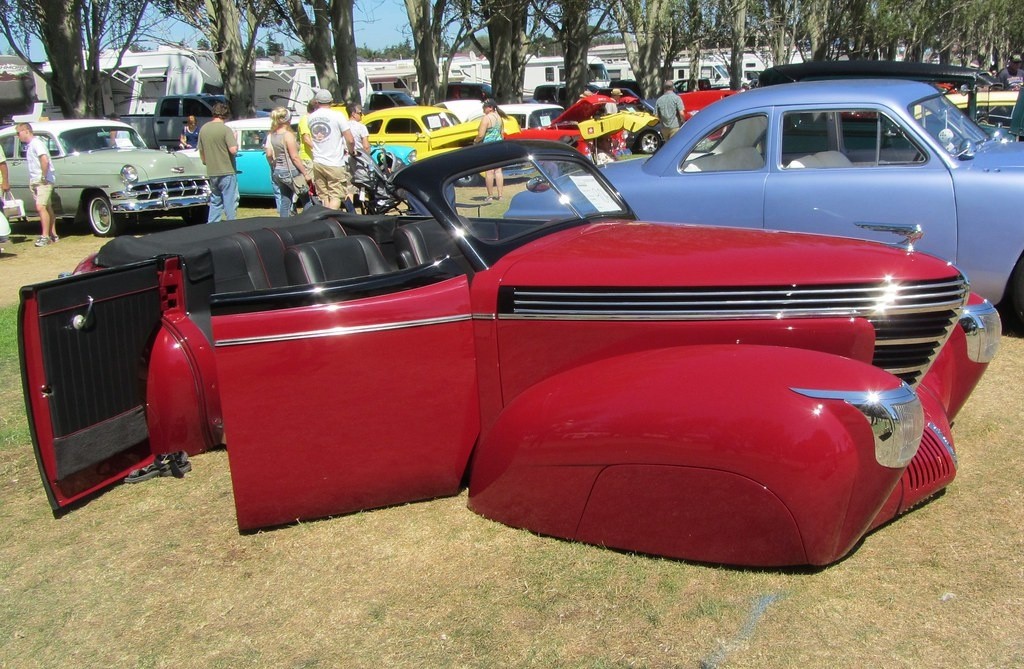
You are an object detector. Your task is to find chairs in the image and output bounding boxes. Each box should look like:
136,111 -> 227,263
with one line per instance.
390,213 -> 481,270
789,151 -> 852,168
283,235 -> 390,286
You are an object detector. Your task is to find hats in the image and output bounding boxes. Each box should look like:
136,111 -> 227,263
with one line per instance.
315,89 -> 332,104
482,98 -> 496,108
611,88 -> 623,96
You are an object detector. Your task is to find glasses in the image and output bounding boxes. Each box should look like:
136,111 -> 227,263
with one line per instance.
357,112 -> 362,115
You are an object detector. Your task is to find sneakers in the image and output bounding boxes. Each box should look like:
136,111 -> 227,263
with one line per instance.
50,235 -> 59,243
35,236 -> 52,246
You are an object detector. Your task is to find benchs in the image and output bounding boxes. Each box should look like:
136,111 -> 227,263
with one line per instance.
682,146 -> 765,173
191,216 -> 349,294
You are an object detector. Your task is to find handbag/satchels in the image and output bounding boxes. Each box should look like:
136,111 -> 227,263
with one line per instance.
4,198 -> 25,218
3,206 -> 20,217
293,173 -> 310,194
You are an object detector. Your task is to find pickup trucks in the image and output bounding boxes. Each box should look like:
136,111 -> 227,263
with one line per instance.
120,93 -> 271,151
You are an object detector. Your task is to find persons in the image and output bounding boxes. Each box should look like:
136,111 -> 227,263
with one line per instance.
16,123 -> 59,247
606,88 -> 623,114
342,102 -> 388,206
990,55 -> 1024,91
0,145 -> 11,243
298,89 -> 354,210
266,106 -> 312,218
655,80 -> 686,143
474,98 -> 509,202
180,115 -> 200,149
198,102 -> 241,225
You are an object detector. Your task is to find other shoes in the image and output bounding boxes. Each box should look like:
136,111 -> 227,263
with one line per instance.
485,197 -> 493,202
498,196 -> 505,202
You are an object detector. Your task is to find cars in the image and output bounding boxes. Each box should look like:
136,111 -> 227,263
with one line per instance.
0,117 -> 211,237
505,79 -> 1024,341
331,61 -> 1023,182
17,138 -> 1001,572
174,115 -> 416,206
